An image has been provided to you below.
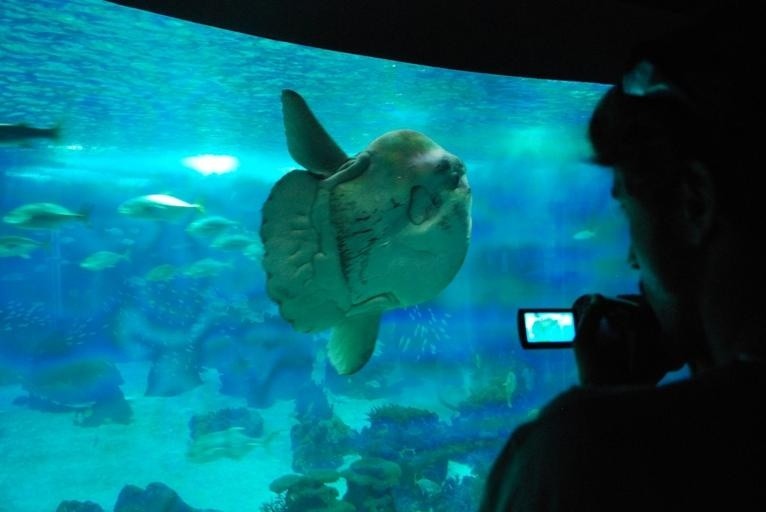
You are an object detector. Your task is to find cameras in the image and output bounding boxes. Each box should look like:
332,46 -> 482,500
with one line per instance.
517,307 -> 581,350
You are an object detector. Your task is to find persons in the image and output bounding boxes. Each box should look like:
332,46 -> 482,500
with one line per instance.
480,42 -> 766,511
